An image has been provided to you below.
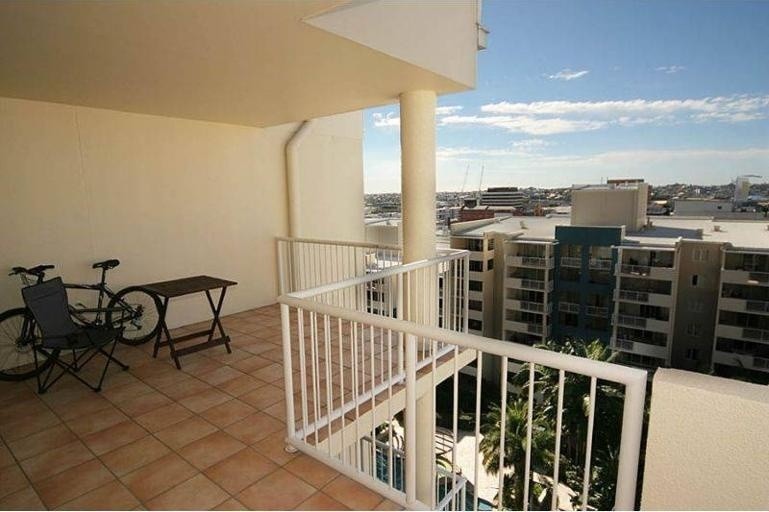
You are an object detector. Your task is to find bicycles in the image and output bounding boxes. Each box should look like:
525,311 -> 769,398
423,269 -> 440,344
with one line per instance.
0,258 -> 164,383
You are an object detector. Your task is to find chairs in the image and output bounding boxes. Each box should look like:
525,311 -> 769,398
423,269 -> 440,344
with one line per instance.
20,276 -> 130,394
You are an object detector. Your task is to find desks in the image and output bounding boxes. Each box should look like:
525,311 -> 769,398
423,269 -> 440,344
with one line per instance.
139,275 -> 238,369
